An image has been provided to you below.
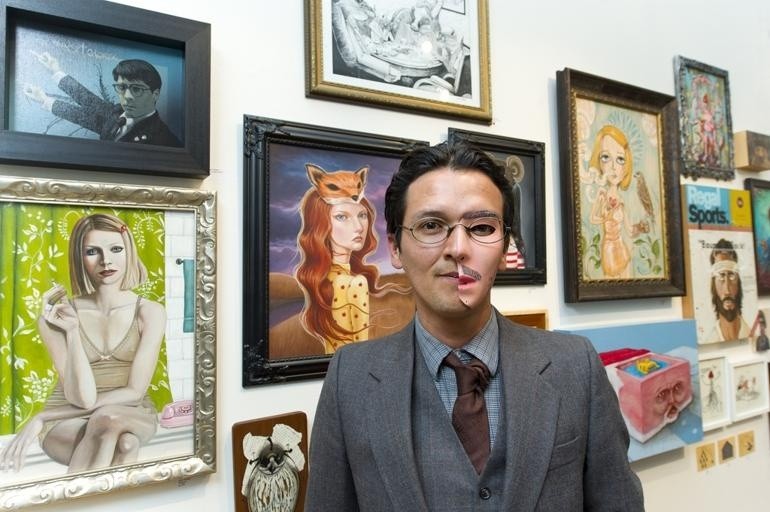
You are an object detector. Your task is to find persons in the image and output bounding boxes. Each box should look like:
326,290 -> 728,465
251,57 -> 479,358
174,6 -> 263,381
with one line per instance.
292,186 -> 416,355
699,238 -> 752,345
302,131 -> 647,511
18,49 -> 183,150
0,211 -> 170,479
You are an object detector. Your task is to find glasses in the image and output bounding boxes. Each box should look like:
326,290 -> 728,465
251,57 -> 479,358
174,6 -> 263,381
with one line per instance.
112,84 -> 151,96
397,217 -> 512,243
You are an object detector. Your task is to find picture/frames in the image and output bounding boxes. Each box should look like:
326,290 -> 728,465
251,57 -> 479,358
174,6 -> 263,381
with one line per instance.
0,0 -> 211,179
0,173 -> 217,512
732,362 -> 768,418
673,53 -> 734,181
556,67 -> 686,305
241,113 -> 431,390
303,0 -> 492,129
448,127 -> 548,285
504,311 -> 548,333
697,356 -> 727,427
744,177 -> 770,297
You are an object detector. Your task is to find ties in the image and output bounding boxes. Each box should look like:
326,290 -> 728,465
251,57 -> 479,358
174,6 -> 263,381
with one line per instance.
446,351 -> 491,475
111,117 -> 126,137
